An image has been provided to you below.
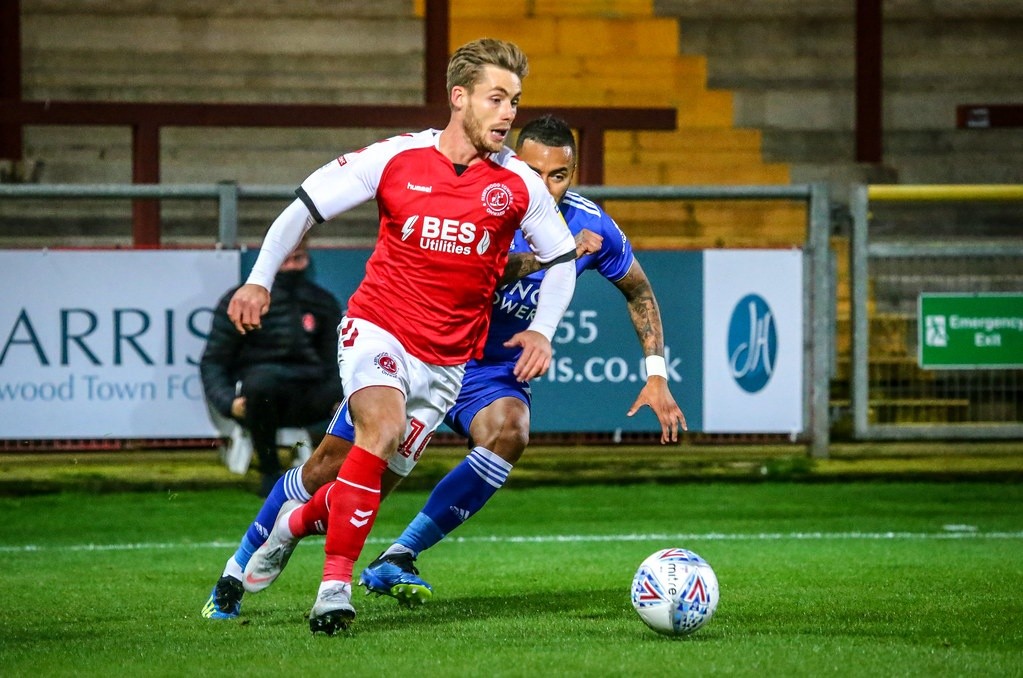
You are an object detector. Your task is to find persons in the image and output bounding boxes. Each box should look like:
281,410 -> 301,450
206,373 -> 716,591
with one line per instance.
200,116 -> 688,620
201,237 -> 342,500
226,36 -> 576,637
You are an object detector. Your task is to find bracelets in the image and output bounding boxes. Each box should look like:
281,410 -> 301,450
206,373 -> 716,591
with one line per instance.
645,355 -> 668,380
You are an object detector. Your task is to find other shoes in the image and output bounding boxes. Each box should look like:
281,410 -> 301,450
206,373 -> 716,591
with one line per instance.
258,475 -> 279,498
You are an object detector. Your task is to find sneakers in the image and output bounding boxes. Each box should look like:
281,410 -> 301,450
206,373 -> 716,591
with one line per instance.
202,575 -> 244,620
359,551 -> 435,609
242,499 -> 304,595
308,580 -> 356,636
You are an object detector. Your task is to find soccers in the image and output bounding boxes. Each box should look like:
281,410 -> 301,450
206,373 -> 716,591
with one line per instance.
631,548 -> 721,635
